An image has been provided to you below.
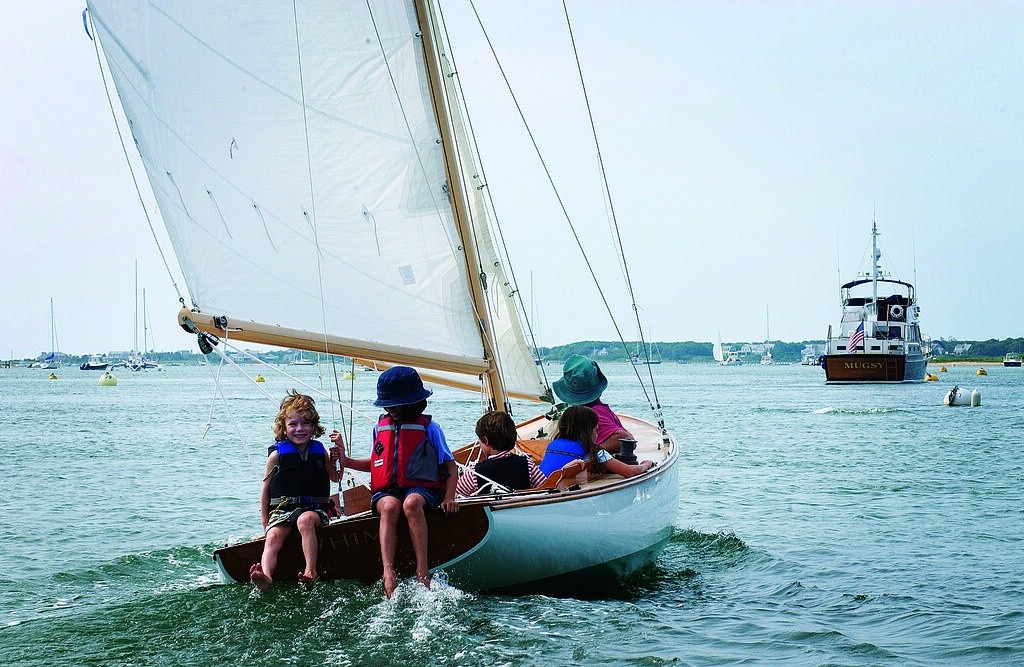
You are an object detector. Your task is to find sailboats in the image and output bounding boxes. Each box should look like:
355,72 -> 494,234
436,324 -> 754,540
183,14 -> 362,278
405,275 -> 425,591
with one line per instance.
26,297 -> 61,369
82,0 -> 679,595
713,329 -> 744,366
81,257 -> 163,372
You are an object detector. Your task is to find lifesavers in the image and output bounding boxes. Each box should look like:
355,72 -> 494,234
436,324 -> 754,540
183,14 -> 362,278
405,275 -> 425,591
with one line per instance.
890,305 -> 904,318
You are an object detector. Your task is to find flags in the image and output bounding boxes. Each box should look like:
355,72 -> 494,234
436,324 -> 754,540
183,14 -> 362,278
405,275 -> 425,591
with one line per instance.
849,321 -> 864,352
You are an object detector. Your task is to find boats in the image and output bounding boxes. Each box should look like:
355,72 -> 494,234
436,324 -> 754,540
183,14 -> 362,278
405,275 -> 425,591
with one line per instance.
761,351 -> 773,365
1003,351 -> 1022,367
818,197 -> 931,384
627,324 -> 662,365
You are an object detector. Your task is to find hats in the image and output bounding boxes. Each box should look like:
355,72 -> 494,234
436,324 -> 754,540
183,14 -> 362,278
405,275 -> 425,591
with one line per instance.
552,354 -> 608,405
372,366 -> 433,407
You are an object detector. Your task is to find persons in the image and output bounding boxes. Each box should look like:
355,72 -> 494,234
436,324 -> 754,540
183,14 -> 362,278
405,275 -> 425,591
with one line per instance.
552,356 -> 636,453
330,367 -> 459,599
250,388 -> 344,591
454,411 -> 584,496
539,407 -> 653,478
